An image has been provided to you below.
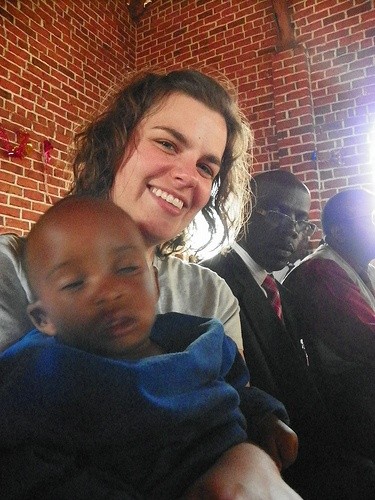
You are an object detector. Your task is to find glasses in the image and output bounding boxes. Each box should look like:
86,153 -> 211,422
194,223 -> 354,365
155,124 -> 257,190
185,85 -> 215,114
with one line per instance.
253,207 -> 316,237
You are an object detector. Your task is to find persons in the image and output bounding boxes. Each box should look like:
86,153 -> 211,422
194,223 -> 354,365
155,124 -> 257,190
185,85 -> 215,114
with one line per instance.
281,188 -> 375,460
0,194 -> 298,500
0,70 -> 303,500
196,170 -> 375,499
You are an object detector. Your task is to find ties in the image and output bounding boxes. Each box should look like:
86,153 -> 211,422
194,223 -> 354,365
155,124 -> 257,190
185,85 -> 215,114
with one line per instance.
262,276 -> 282,321
